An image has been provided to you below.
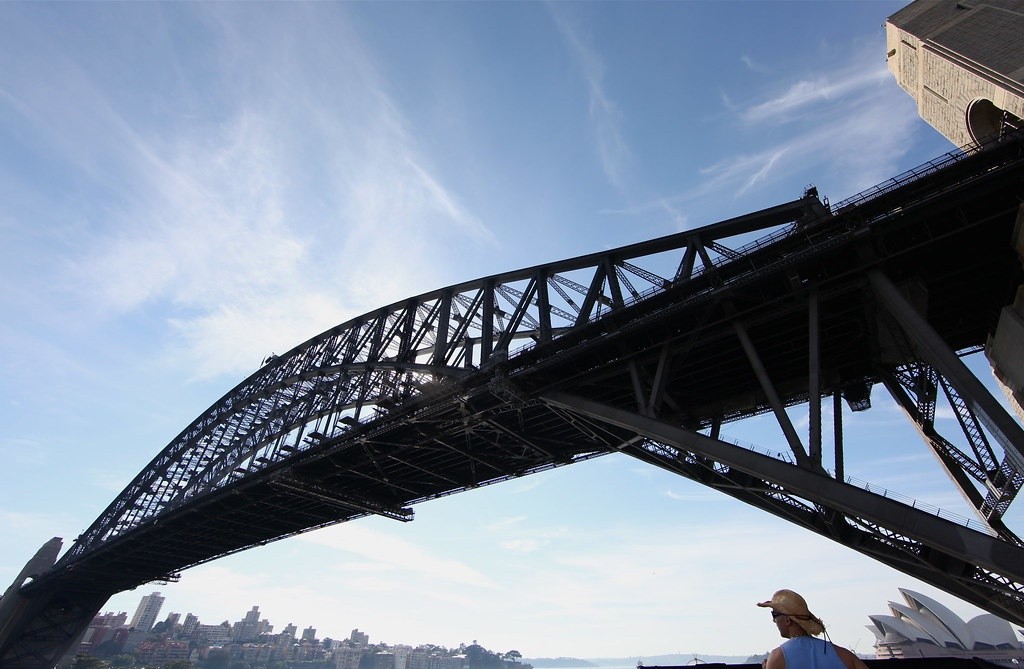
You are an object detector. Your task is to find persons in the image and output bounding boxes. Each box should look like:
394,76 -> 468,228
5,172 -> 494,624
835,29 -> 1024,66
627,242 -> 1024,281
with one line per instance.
757,588 -> 865,669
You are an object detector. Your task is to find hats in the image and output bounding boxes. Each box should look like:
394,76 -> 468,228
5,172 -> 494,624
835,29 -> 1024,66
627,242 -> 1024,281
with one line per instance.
757,589 -> 825,636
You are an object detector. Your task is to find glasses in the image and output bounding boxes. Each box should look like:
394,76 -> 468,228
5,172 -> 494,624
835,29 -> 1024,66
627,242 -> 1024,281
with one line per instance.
771,611 -> 783,620
